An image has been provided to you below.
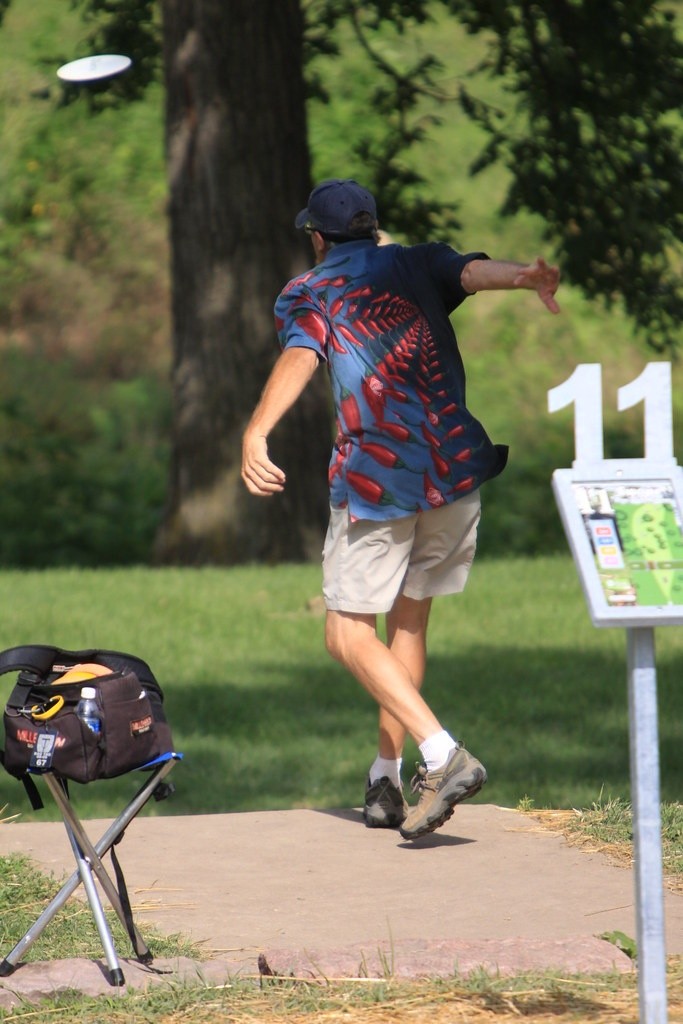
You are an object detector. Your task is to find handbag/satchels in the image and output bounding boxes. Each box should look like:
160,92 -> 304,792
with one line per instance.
0,645 -> 183,801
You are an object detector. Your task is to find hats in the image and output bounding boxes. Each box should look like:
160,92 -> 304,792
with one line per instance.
294,178 -> 377,234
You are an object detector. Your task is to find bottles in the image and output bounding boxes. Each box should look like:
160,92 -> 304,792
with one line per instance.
76,687 -> 100,733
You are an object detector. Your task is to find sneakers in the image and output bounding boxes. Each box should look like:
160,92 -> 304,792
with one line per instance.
399,739 -> 488,840
363,776 -> 408,828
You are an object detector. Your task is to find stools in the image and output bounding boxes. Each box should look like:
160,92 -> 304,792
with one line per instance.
1,751 -> 185,987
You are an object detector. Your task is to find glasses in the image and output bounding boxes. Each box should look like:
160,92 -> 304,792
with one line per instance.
304,222 -> 316,234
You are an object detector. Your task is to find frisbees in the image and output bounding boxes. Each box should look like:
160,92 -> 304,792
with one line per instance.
56,53 -> 132,81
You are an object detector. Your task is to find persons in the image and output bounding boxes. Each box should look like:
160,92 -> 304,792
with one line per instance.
238,180 -> 561,841
